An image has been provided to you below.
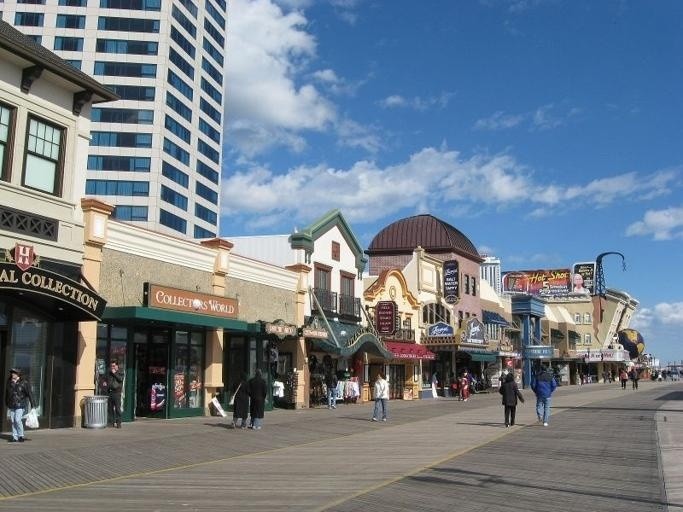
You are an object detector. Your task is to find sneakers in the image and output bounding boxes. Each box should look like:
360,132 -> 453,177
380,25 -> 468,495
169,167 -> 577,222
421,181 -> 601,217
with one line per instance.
230,421 -> 262,430
328,405 -> 336,409
372,418 -> 387,422
8,436 -> 25,442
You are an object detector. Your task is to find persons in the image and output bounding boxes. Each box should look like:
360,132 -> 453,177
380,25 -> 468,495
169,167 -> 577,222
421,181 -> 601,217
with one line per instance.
448,369 -> 477,402
323,367 -> 339,410
228,372 -> 250,431
98,360 -> 125,429
371,371 -> 389,422
498,372 -> 524,428
244,368 -> 268,431
529,364 -> 557,427
3,366 -> 37,444
497,371 -> 507,385
572,365 -> 682,392
430,371 -> 440,399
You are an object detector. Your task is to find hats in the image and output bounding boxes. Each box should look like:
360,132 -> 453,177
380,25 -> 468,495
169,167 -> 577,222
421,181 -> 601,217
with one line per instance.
8,367 -> 24,375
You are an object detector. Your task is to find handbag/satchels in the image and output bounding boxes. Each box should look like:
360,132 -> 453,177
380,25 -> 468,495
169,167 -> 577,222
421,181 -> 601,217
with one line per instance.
228,396 -> 235,406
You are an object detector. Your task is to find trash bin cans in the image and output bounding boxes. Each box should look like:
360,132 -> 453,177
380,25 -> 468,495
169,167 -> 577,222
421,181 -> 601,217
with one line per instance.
83,396 -> 109,428
